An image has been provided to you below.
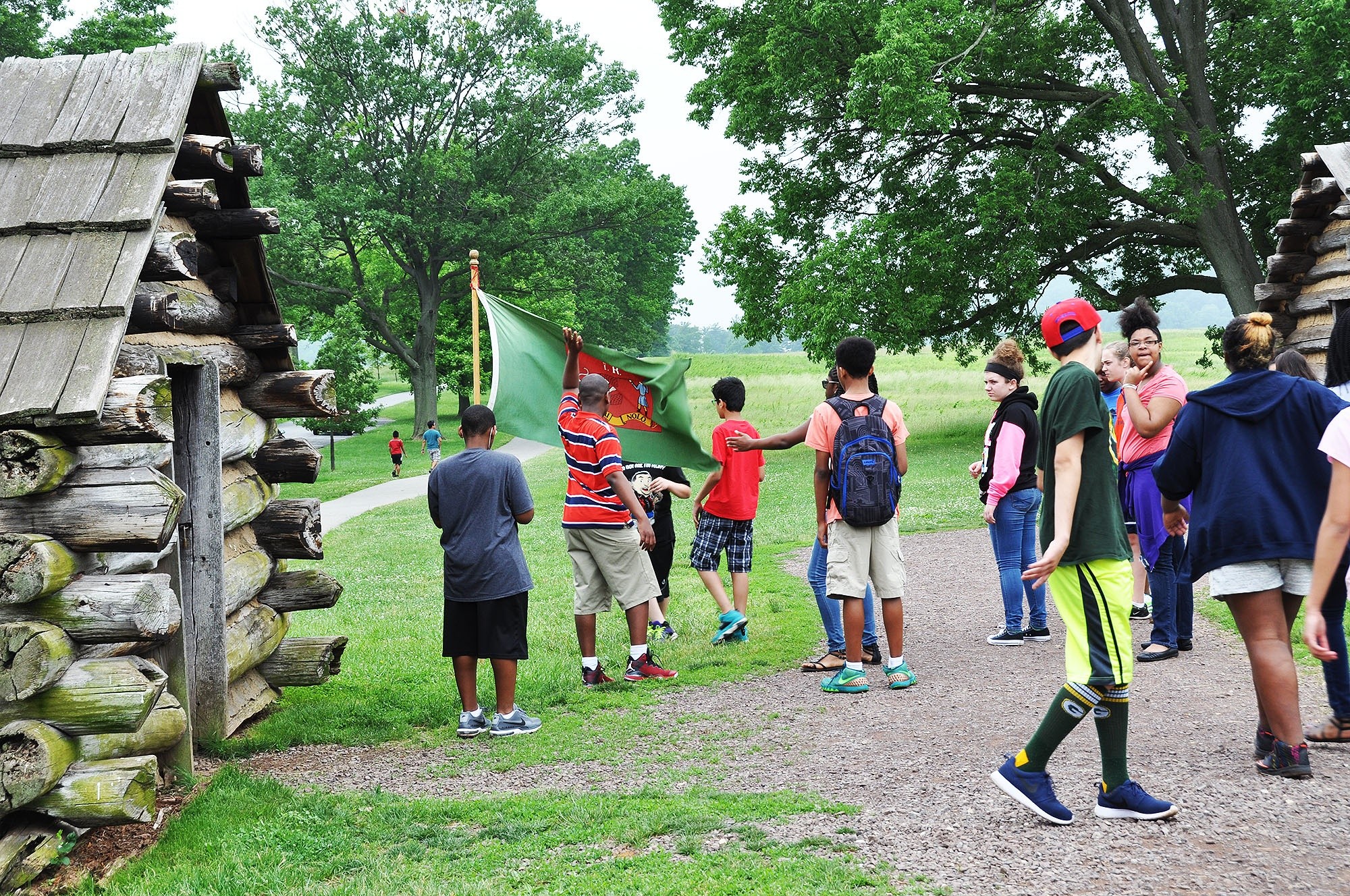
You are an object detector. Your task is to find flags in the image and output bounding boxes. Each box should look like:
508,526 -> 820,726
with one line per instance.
475,289 -> 722,471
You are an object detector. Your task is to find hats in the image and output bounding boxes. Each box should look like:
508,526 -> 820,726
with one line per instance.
1041,298 -> 1102,349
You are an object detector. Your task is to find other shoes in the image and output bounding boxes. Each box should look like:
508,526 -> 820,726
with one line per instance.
1136,647 -> 1178,662
1140,638 -> 1193,652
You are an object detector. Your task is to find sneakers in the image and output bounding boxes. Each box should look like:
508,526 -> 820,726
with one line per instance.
1129,603 -> 1152,619
1021,624 -> 1051,641
1094,780 -> 1177,820
1254,724 -> 1276,756
648,621 -> 678,646
882,660 -> 917,689
490,707 -> 542,735
710,610 -> 748,646
724,626 -> 748,642
990,755 -> 1074,825
1253,738 -> 1311,775
456,711 -> 492,736
622,652 -> 678,682
820,662 -> 869,694
986,628 -> 1024,646
581,662 -> 615,687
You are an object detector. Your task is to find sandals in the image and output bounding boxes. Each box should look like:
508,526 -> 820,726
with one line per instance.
861,642 -> 882,666
800,649 -> 850,671
1302,716 -> 1350,742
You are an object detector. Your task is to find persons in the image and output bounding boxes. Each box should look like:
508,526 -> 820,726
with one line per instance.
968,338 -> 1051,647
421,420 -> 442,474
428,404 -> 542,738
689,376 -> 764,647
1094,297 -> 1189,662
619,460 -> 692,643
1152,311 -> 1350,779
990,298 -> 1178,824
558,324 -> 678,687
1278,304 -> 1350,744
388,430 -> 408,477
726,335 -> 917,694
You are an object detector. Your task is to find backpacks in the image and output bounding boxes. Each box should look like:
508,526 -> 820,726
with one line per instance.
823,394 -> 903,528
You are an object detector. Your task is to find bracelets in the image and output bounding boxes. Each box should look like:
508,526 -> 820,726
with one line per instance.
421,449 -> 424,451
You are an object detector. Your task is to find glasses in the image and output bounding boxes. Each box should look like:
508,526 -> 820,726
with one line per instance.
1129,340 -> 1161,347
